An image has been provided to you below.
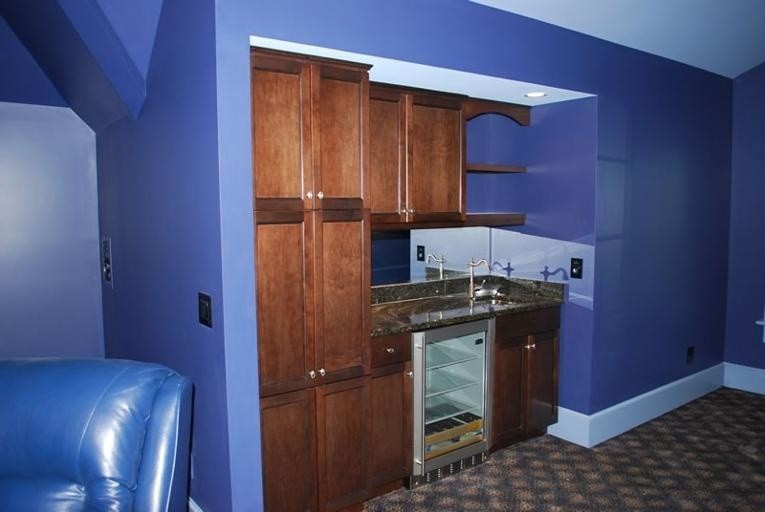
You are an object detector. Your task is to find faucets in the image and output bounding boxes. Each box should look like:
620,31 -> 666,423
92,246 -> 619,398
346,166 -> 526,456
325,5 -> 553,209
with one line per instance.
468,299 -> 477,315
425,253 -> 447,281
425,310 -> 443,324
467,258 -> 492,298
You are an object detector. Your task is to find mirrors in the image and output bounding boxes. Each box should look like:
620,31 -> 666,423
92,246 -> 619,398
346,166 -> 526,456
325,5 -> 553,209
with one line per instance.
370,226 -> 491,288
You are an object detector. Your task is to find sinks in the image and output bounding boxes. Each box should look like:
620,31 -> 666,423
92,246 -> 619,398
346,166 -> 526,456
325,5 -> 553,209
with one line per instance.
473,284 -> 506,300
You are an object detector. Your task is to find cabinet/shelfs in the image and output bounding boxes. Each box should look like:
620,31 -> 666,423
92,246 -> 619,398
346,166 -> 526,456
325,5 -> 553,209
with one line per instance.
368,81 -> 466,229
489,304 -> 560,454
253,210 -> 371,399
250,45 -> 373,211
463,97 -> 532,228
370,332 -> 412,500
258,375 -> 370,512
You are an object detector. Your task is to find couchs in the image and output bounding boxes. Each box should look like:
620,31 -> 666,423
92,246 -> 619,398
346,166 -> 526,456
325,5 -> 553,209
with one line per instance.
0,358 -> 193,512
